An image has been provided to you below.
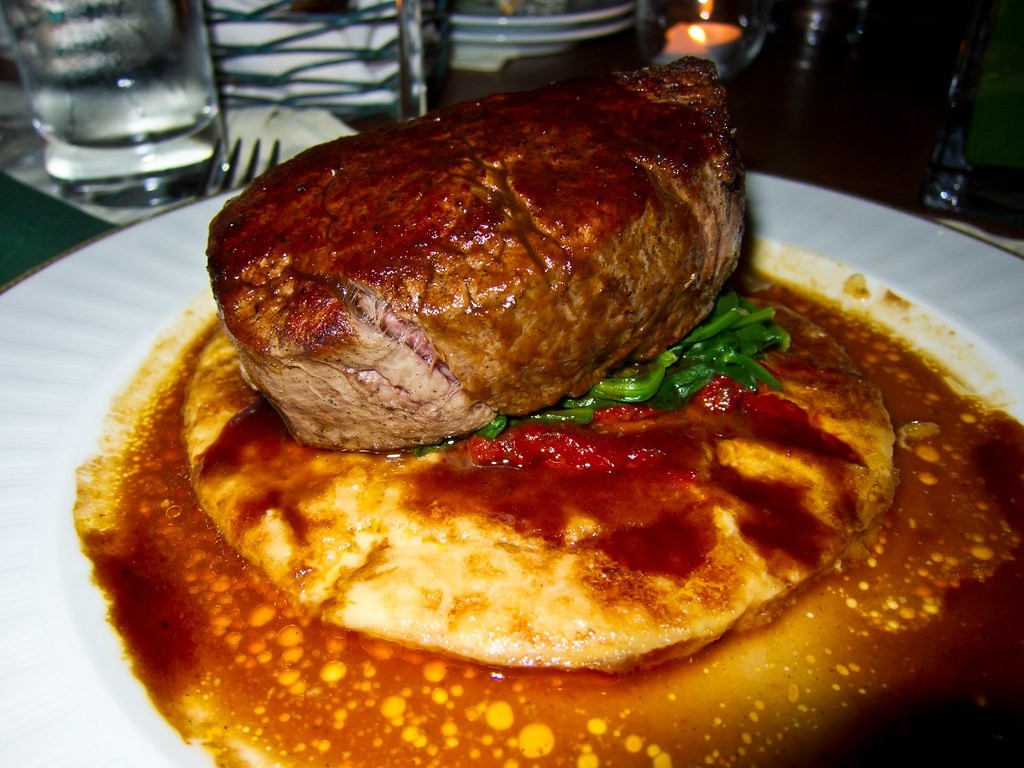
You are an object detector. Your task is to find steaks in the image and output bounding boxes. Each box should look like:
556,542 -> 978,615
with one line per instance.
205,56 -> 747,449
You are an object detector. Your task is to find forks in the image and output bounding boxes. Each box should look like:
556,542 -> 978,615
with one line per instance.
203,136 -> 279,198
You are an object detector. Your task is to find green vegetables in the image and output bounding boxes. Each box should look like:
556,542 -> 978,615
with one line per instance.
473,284 -> 792,440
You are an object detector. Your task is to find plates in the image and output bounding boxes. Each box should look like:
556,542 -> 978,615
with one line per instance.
0,167 -> 1024,768
446,0 -> 633,57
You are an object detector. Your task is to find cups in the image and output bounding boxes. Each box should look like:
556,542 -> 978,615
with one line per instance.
638,0 -> 769,74
921,0 -> 1024,227
0,0 -> 223,207
